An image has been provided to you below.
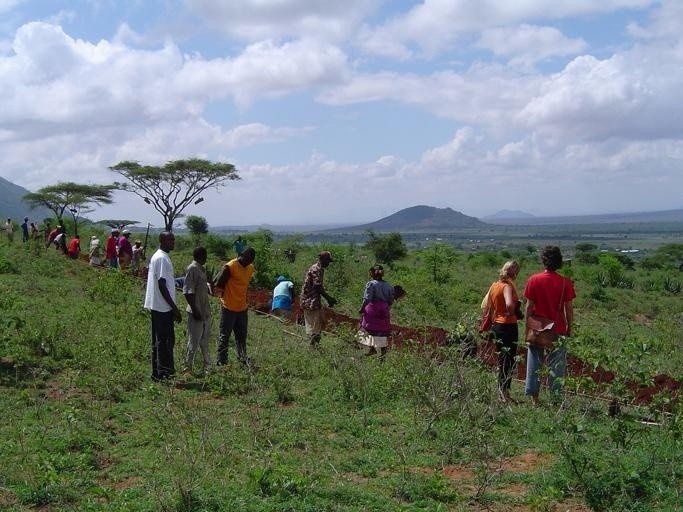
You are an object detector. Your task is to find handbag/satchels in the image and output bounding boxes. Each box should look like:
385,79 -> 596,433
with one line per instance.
525,278 -> 565,348
478,306 -> 491,334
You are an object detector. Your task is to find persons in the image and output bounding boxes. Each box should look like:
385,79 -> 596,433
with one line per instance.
523,249 -> 577,410
106,230 -> 117,269
115,230 -> 120,245
119,229 -> 133,270
89,236 -> 102,267
68,235 -> 81,259
214,247 -> 255,368
354,264 -> 396,360
47,225 -> 61,248
54,233 -> 67,254
132,240 -> 146,276
272,275 -> 294,317
479,262 -> 524,406
144,231 -> 182,383
181,247 -> 212,376
300,250 -> 338,344
233,236 -> 244,256
31,223 -> 38,241
39,224 -> 50,241
6,217 -> 13,242
21,216 -> 29,243
59,220 -> 65,233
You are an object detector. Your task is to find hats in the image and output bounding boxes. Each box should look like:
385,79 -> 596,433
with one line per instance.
317,251 -> 337,263
111,228 -> 119,233
122,230 -> 132,235
277,275 -> 287,283
